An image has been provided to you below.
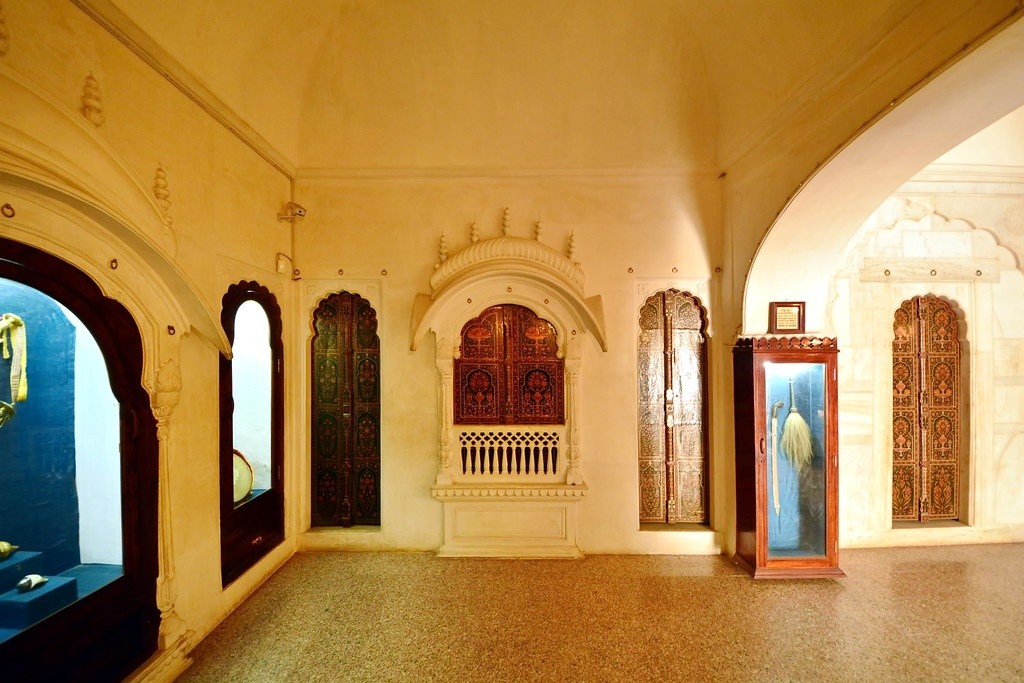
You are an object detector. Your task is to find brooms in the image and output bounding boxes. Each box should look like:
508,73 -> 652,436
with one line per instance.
779,379 -> 814,473
